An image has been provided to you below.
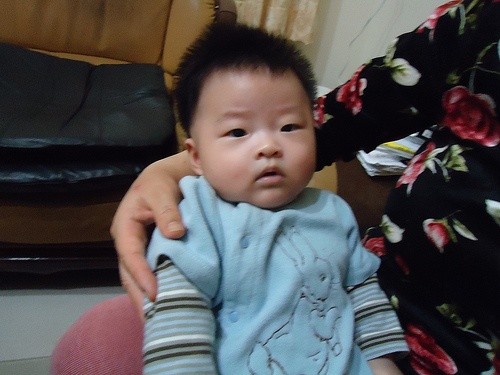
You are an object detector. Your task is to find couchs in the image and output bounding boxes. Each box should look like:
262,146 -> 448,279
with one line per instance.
0,0 -> 397,285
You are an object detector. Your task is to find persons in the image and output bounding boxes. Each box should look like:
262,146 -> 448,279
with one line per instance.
49,0 -> 500,375
143,21 -> 411,375
